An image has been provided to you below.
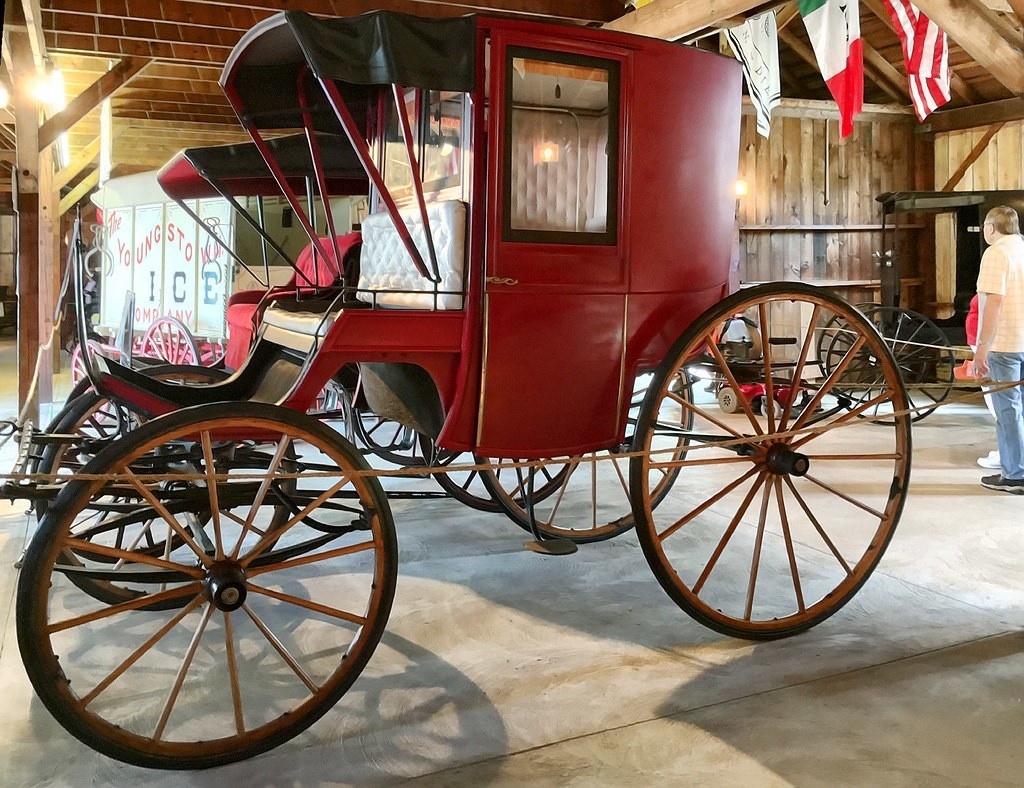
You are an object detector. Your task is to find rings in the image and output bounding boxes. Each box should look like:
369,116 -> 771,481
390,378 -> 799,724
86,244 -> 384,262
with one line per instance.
976,370 -> 978,374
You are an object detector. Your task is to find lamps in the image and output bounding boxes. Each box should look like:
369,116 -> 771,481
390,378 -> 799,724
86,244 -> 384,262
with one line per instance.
534,140 -> 561,164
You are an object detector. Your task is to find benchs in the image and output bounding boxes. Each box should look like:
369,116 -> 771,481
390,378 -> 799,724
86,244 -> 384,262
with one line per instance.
225,233 -> 362,327
265,198 -> 467,351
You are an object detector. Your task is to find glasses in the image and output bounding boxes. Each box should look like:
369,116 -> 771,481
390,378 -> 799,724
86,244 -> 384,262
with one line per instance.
980,222 -> 991,231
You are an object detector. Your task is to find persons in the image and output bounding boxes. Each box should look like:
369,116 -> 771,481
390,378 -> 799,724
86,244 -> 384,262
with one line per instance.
970,205 -> 1024,495
965,293 -> 1005,469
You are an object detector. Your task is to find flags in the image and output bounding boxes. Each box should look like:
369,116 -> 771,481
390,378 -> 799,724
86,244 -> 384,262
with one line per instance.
725,10 -> 782,140
796,0 -> 864,138
881,0 -> 950,124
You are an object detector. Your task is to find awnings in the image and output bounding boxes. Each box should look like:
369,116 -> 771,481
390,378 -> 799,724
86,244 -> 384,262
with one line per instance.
217,11 -> 476,288
156,132 -> 371,284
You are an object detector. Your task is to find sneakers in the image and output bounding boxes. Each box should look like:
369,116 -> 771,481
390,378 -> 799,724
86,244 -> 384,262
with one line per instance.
978,449 -> 1002,469
981,474 -> 1024,495
